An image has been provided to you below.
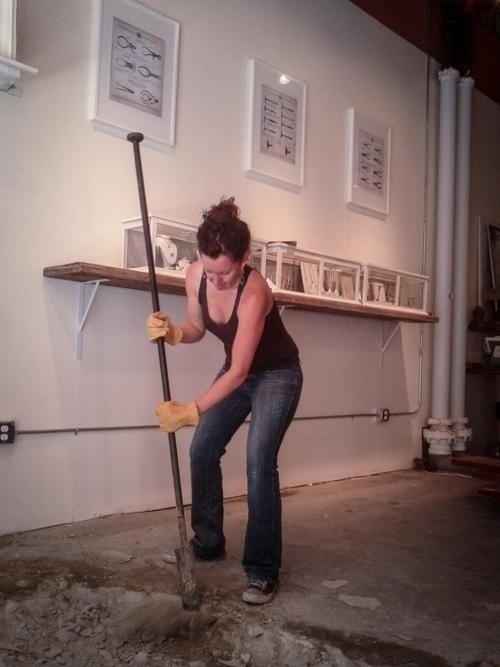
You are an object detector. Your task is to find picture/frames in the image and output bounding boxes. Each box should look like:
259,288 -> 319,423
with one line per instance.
343,108 -> 392,220
89,0 -> 181,148
243,56 -> 307,189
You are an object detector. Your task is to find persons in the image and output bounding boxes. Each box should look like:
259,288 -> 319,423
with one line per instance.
141,196 -> 309,609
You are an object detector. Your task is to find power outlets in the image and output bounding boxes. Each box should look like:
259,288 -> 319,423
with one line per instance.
0,421 -> 14,443
377,408 -> 390,423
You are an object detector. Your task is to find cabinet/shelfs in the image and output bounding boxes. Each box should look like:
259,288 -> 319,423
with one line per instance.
44,215 -> 439,369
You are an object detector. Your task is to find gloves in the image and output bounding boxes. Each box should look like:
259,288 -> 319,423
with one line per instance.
147,312 -> 183,346
157,401 -> 199,433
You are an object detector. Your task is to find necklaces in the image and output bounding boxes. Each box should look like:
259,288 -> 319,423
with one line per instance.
301,261 -> 320,291
163,240 -> 176,258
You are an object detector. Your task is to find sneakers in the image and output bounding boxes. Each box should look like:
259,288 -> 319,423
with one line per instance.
242,577 -> 278,604
164,550 -> 225,564
